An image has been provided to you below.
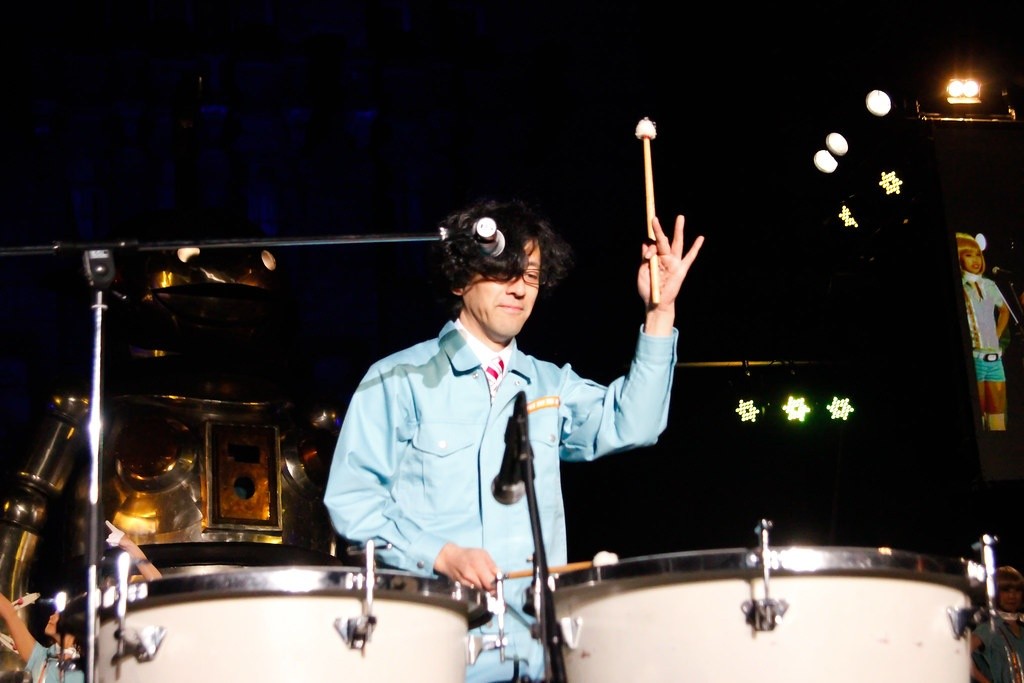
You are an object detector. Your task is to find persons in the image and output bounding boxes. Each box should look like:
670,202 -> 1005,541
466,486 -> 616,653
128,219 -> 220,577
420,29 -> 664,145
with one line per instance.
971,566 -> 1024,683
956,233 -> 1010,431
104,518 -> 164,583
0,594 -> 85,683
322,191 -> 705,683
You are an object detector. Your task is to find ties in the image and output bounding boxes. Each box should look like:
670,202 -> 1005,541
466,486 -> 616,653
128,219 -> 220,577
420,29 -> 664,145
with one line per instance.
486,357 -> 504,388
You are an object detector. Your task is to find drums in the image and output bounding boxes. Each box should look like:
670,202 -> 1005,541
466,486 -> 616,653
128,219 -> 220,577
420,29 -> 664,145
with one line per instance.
53,562 -> 522,683
542,539 -> 978,683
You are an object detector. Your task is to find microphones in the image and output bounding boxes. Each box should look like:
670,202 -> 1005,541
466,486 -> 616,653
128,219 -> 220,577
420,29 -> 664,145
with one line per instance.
491,392 -> 528,505
471,216 -> 506,258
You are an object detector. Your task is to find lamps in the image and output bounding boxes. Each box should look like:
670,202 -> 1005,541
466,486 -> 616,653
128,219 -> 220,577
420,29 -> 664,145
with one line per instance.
944,78 -> 983,104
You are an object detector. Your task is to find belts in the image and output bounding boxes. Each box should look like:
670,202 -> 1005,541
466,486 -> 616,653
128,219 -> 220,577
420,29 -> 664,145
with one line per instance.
973,348 -> 1002,361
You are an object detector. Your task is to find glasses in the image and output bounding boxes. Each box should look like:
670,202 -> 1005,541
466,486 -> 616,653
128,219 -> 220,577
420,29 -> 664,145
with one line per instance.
522,269 -> 549,285
